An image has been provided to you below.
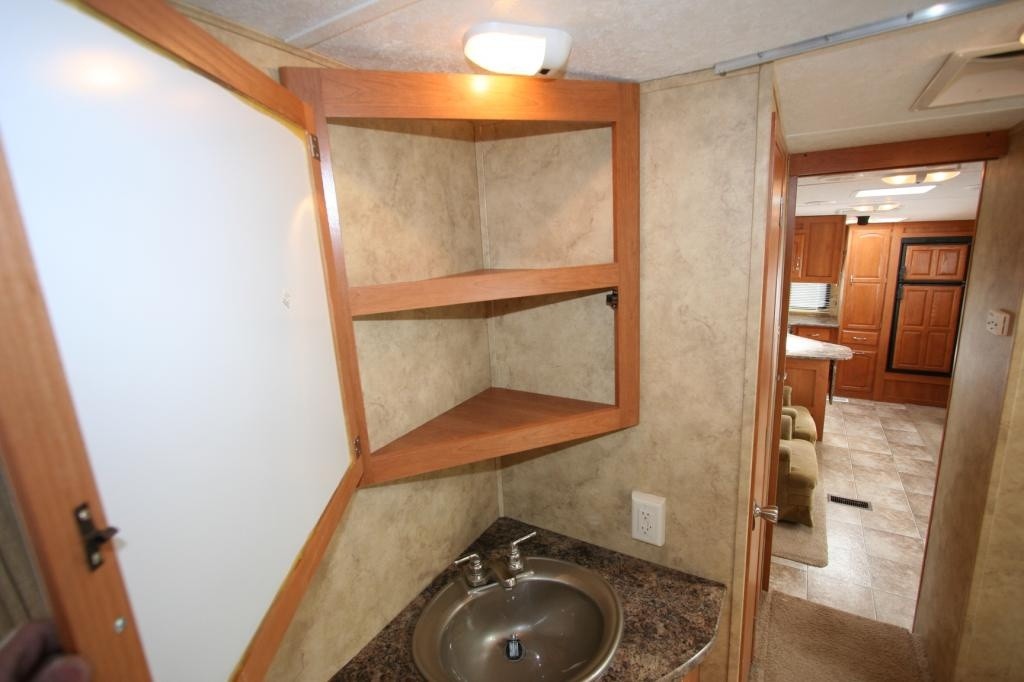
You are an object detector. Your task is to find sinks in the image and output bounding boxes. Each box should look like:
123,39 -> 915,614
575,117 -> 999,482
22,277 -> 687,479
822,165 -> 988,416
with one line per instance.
439,576 -> 623,682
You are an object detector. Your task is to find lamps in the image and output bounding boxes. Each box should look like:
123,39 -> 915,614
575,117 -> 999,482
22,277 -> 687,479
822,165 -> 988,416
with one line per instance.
883,165 -> 962,186
462,18 -> 573,80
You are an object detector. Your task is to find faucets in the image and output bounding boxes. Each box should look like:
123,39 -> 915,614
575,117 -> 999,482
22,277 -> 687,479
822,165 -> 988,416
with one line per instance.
488,561 -> 517,591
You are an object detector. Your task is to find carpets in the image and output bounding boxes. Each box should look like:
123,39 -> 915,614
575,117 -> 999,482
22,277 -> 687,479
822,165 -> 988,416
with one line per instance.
748,588 -> 935,682
772,441 -> 828,567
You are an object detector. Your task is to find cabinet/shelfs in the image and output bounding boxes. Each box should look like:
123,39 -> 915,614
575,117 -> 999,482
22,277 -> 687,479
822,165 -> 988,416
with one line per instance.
277,64 -> 641,490
783,215 -> 978,442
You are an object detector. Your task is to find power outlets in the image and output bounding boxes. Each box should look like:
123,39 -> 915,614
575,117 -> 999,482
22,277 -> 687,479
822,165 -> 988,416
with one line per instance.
631,490 -> 666,547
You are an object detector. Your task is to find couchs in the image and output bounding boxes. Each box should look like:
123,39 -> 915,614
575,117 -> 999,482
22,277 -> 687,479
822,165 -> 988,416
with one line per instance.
775,385 -> 818,527
